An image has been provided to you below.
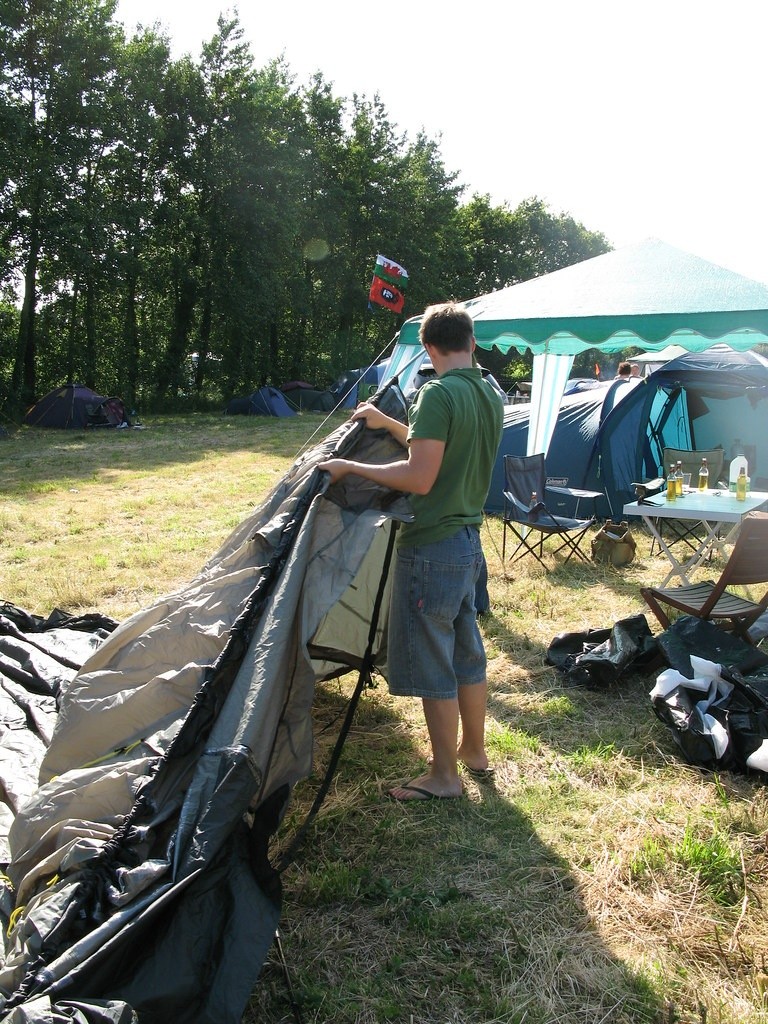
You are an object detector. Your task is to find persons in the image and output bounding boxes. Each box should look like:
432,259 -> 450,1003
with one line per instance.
315,302 -> 504,800
631,363 -> 640,377
614,361 -> 631,380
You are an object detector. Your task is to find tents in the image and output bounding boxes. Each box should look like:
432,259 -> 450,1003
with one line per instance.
21,383 -> 132,429
224,235 -> 768,527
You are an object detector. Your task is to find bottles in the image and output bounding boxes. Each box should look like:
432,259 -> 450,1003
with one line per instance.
736,467 -> 746,500
530,492 -> 537,509
698,457 -> 709,491
666,464 -> 676,502
675,461 -> 683,496
729,454 -> 750,498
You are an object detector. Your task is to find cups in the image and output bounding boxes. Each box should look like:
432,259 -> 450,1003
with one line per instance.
682,472 -> 692,494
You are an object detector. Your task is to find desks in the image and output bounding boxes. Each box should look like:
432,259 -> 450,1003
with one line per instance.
623,487 -> 768,614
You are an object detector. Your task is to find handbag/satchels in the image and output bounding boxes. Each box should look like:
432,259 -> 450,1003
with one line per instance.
591,519 -> 636,565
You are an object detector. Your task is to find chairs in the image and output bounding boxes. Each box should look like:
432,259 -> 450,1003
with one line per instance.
630,447 -> 727,563
501,452 -> 605,572
640,511 -> 768,647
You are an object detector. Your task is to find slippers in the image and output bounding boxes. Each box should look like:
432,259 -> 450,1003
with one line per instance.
384,777 -> 467,802
457,753 -> 494,775
